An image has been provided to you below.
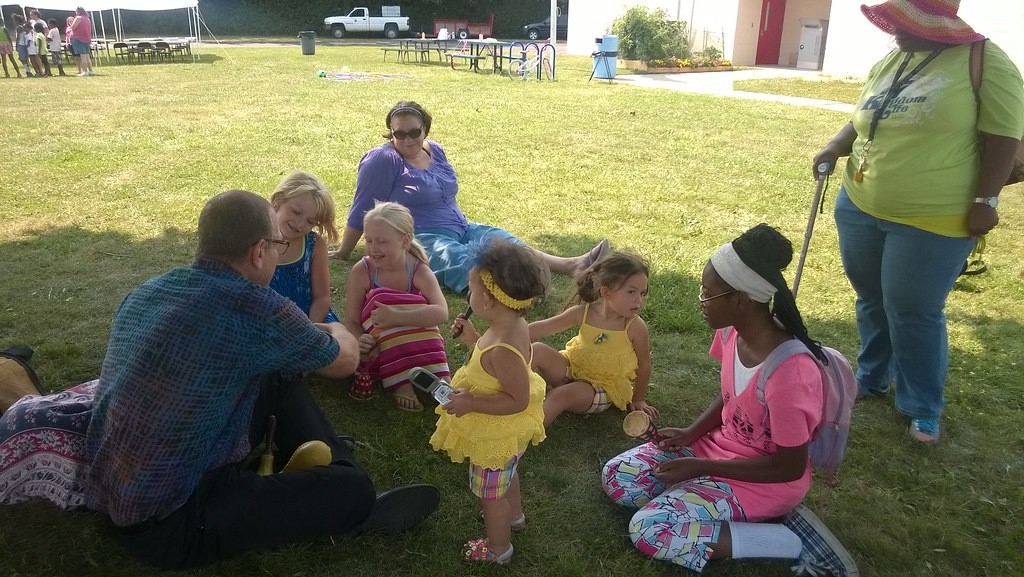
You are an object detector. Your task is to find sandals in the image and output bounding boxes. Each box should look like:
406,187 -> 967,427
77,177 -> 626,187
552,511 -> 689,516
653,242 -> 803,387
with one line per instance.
460,538 -> 513,565
478,509 -> 525,532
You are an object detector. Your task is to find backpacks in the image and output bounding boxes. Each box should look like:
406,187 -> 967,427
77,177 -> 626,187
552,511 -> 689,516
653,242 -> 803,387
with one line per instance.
719,324 -> 859,480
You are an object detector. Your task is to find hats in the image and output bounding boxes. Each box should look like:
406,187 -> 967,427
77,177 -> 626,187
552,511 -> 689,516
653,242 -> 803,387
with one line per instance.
860,0 -> 985,43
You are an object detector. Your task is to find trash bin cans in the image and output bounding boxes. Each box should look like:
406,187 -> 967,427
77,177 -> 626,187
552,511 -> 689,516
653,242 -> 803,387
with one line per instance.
798,17 -> 830,70
299,31 -> 316,55
593,35 -> 618,78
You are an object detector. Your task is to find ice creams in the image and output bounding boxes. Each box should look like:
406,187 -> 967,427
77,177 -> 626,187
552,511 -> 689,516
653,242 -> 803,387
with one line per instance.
623,410 -> 659,442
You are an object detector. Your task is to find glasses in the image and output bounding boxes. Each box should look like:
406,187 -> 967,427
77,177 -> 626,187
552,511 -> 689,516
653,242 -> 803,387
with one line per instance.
252,230 -> 290,255
699,285 -> 753,310
391,124 -> 424,140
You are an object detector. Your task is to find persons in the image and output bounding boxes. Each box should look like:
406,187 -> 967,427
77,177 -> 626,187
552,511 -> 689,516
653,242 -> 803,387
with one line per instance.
85,190 -> 440,570
601,224 -> 859,577
269,170 -> 340,325
65,6 -> 95,77
427,238 -> 547,565
812,0 -> 1024,446
13,10 -> 66,77
0,17 -> 22,78
328,100 -> 609,298
344,202 -> 452,412
528,251 -> 660,428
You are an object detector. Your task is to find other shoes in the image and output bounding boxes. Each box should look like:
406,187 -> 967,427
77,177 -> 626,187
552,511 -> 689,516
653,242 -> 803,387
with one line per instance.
78,71 -> 93,76
360,484 -> 440,533
24,70 -> 66,76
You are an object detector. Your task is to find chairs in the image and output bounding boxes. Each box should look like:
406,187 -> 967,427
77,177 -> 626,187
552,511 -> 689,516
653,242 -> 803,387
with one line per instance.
46,39 -> 190,64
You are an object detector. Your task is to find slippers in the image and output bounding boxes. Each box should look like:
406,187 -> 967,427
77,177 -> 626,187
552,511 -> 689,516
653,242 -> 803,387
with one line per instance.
540,260 -> 551,304
577,238 -> 607,275
391,388 -> 425,412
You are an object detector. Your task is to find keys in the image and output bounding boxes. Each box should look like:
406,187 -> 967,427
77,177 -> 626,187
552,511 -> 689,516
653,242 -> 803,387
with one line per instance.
857,157 -> 865,173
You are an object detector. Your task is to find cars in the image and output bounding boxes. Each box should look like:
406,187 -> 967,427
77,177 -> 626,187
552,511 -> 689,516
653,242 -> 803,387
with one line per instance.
522,15 -> 567,40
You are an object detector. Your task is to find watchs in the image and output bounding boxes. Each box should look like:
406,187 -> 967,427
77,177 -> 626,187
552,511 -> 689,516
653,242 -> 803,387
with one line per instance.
974,197 -> 999,208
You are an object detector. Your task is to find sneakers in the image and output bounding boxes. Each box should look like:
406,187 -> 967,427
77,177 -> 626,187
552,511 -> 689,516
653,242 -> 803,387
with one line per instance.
910,415 -> 939,442
854,373 -> 891,403
780,505 -> 859,577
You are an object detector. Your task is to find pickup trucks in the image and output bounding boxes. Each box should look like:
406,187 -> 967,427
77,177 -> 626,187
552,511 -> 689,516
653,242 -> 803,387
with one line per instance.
324,7 -> 410,39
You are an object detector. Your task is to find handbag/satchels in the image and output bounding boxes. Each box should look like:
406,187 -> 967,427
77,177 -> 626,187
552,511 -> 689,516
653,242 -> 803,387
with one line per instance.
969,37 -> 1024,185
0,345 -> 45,416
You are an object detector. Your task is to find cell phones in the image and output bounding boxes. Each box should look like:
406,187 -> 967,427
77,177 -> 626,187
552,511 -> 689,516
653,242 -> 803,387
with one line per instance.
408,368 -> 457,405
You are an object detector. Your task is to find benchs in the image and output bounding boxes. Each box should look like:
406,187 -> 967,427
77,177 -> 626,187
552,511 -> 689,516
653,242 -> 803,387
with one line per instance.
381,47 -> 522,74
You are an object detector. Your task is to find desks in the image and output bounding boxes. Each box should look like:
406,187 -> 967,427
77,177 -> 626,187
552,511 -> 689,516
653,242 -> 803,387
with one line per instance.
459,40 -> 511,74
391,38 -> 456,65
116,39 -> 189,46
